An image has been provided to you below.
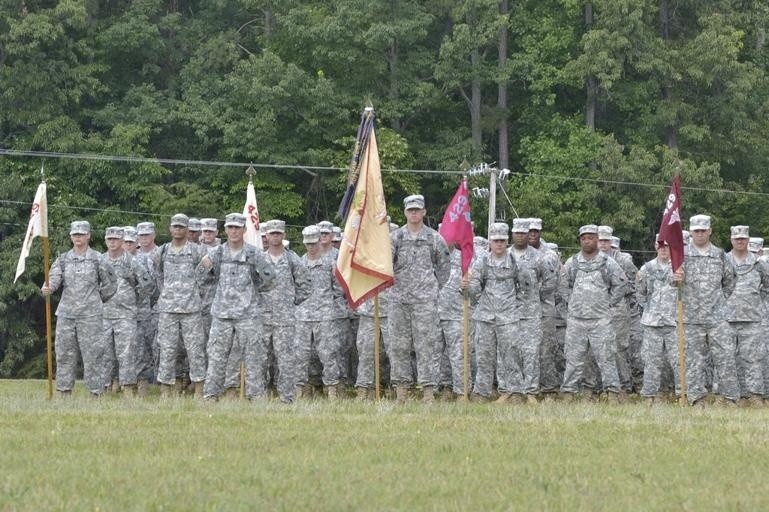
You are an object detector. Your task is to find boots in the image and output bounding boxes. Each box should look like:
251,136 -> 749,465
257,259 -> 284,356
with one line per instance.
249,384 -> 764,408
55,382 -> 148,398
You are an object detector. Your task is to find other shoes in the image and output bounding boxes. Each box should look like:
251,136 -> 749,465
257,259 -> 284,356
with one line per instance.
159,384 -> 232,402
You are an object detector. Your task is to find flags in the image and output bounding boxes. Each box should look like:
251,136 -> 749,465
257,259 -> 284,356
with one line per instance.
334,105 -> 398,311
659,175 -> 689,276
437,176 -> 476,279
242,181 -> 266,255
8,180 -> 51,285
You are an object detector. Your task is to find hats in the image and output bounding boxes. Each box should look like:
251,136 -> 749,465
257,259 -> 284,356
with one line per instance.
730,225 -> 769,254
403,194 -> 426,212
688,215 -> 711,232
69,212 -> 342,247
475,216 -> 622,251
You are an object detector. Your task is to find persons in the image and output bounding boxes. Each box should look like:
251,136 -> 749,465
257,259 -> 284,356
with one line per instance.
42,214 -> 769,409
387,193 -> 452,405
